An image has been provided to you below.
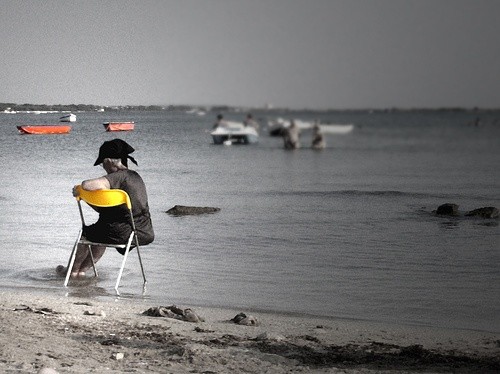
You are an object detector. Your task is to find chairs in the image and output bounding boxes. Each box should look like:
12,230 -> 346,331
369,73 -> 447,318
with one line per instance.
63,184 -> 147,295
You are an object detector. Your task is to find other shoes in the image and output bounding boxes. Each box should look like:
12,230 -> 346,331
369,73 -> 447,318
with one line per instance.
55,265 -> 68,276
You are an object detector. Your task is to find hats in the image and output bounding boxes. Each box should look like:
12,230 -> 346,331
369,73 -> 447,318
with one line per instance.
94,138 -> 138,167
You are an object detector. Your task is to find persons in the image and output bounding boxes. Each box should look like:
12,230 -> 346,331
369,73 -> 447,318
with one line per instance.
56,138 -> 154,278
212,112 -> 325,150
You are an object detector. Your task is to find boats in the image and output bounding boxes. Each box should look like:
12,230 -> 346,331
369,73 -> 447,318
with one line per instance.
60,113 -> 77,122
102,121 -> 135,132
15,124 -> 72,135
267,117 -> 355,135
210,121 -> 258,145
